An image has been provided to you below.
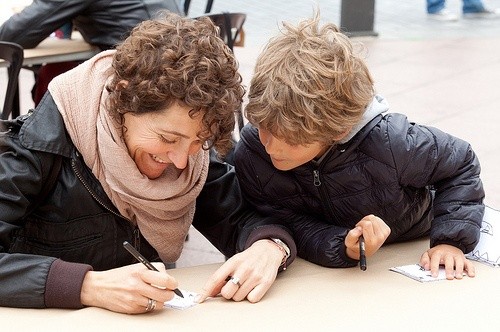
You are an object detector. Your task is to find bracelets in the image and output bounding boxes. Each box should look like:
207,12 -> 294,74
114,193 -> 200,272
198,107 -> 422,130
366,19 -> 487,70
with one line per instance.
270,237 -> 291,266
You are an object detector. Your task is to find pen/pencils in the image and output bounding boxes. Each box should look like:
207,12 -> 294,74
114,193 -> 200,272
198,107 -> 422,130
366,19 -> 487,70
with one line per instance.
123,242 -> 186,298
358,235 -> 367,270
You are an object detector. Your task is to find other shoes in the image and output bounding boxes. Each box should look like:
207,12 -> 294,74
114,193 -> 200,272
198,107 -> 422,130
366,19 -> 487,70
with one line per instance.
463,8 -> 500,18
425,7 -> 460,21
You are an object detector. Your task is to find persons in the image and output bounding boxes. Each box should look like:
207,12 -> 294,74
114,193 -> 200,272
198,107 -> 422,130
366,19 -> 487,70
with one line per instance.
0,12 -> 297,315
0,0 -> 182,52
232,19 -> 485,279
426,0 -> 500,22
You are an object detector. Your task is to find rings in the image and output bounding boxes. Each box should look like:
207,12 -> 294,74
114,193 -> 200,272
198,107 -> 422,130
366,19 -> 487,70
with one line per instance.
146,299 -> 156,311
230,277 -> 240,287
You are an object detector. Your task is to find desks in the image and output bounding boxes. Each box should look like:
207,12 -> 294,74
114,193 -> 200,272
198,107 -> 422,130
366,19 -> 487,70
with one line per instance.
0,37 -> 100,118
0,239 -> 500,332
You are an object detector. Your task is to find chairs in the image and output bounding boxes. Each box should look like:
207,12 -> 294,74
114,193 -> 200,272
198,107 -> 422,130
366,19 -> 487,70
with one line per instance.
205,10 -> 245,131
